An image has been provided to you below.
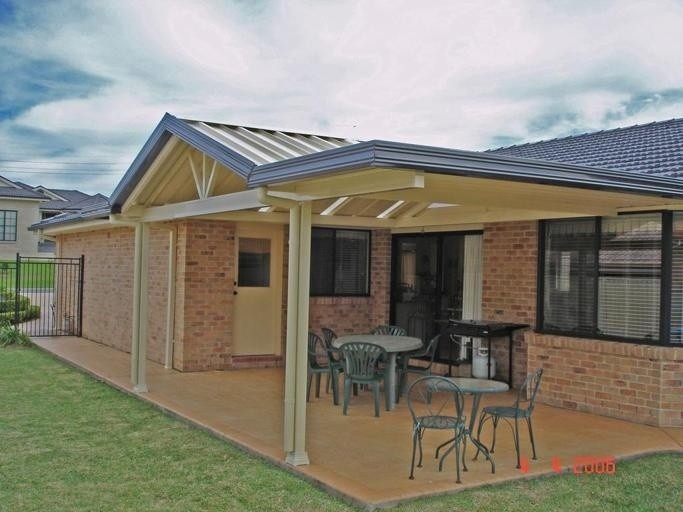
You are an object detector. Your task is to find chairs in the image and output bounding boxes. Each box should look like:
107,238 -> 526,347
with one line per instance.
395,334 -> 442,405
321,327 -> 364,396
369,325 -> 410,385
306,333 -> 346,406
405,376 -> 467,485
473,368 -> 544,468
339,342 -> 390,418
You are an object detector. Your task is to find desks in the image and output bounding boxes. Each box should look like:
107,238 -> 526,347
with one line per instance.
446,321 -> 533,389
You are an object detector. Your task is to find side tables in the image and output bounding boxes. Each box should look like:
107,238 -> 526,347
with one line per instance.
331,332 -> 423,410
424,376 -> 510,461
